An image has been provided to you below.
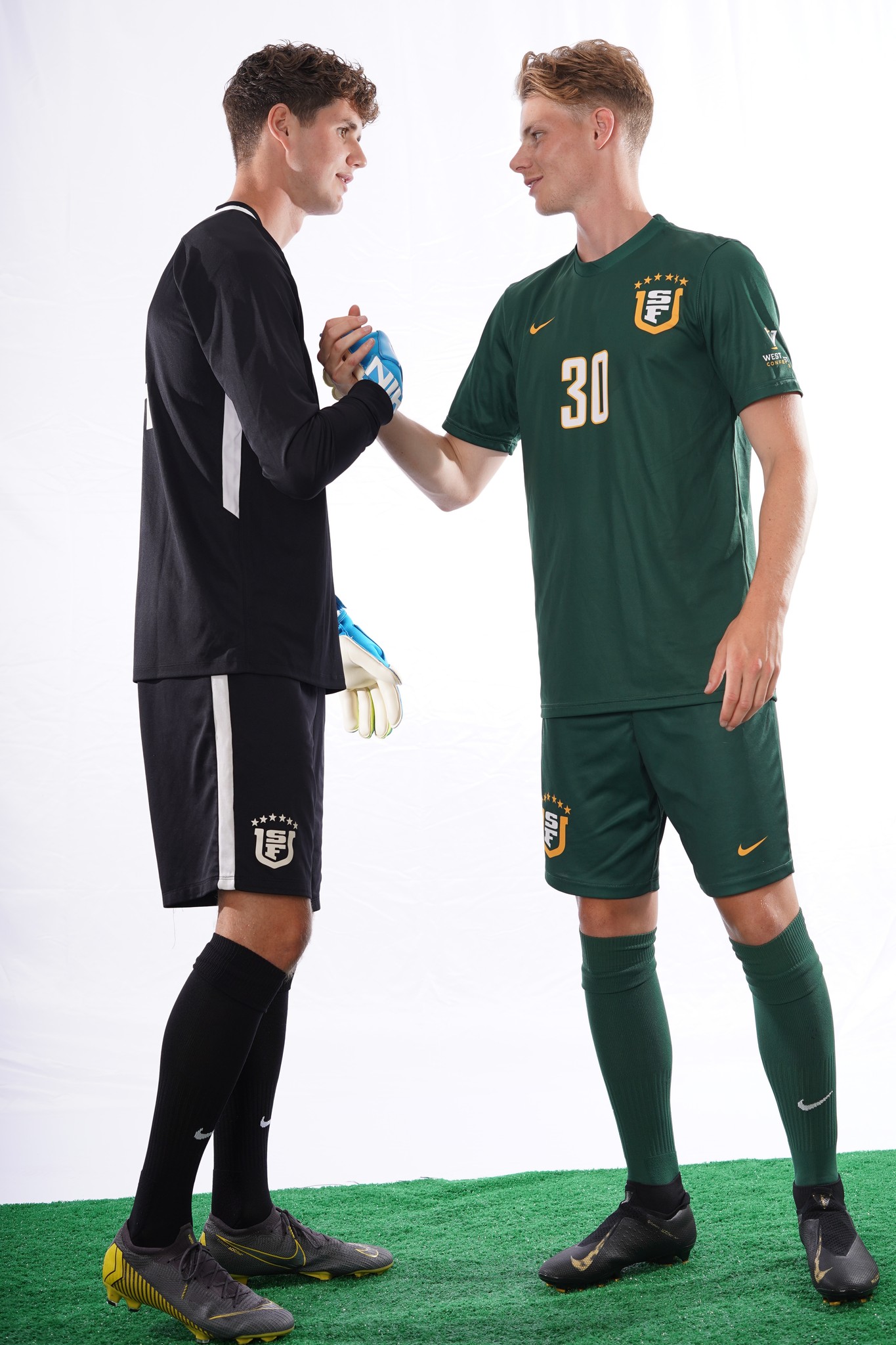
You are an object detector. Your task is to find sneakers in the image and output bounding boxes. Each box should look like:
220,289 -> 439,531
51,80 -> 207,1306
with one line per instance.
792,1176 -> 881,1306
201,1204 -> 396,1289
539,1185 -> 697,1293
105,1221 -> 296,1345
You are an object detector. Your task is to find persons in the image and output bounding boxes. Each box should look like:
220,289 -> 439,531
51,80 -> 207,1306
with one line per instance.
101,42 -> 406,1344
317,36 -> 882,1311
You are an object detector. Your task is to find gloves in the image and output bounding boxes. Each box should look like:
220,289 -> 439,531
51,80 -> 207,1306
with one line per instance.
330,611 -> 405,740
316,327 -> 405,413
